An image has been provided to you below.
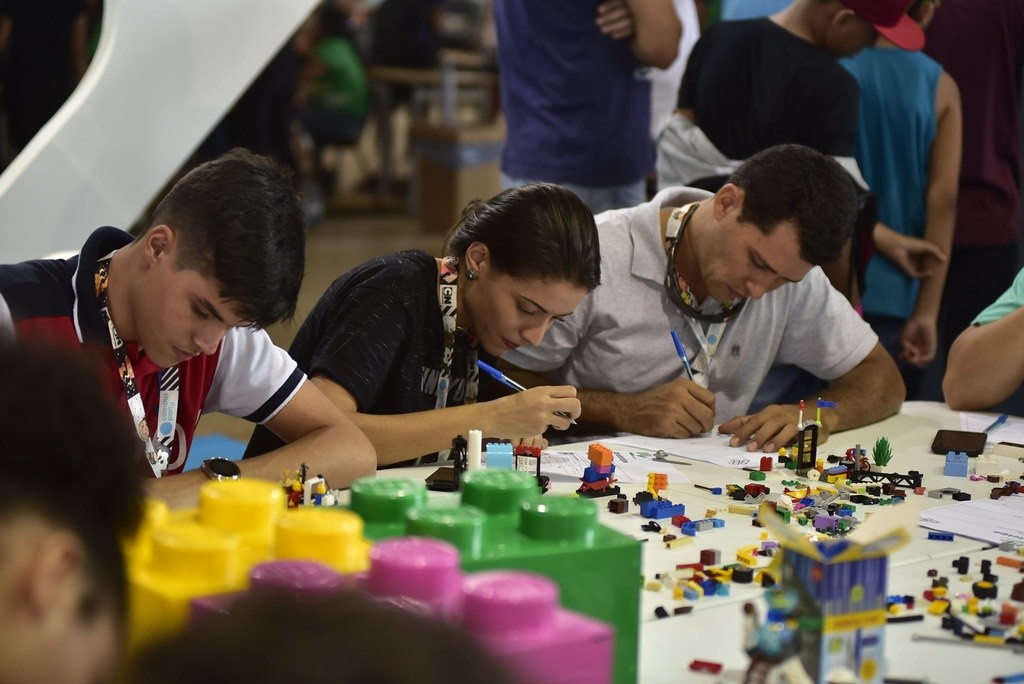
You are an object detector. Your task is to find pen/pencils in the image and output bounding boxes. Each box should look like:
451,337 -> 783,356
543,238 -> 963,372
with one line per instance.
670,329 -> 694,382
983,414 -> 1008,433
476,360 -> 577,425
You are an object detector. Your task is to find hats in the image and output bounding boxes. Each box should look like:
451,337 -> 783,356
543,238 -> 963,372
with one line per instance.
840,0 -> 926,51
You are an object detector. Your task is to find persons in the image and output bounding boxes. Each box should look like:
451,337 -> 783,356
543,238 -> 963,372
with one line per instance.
244,183 -> 601,469
491,0 -> 702,213
0,155 -> 378,489
653,0 -> 925,414
840,0 -> 961,401
492,145 -> 906,446
944,267 -> 1024,412
0,0 -> 436,211
0,334 -> 509,684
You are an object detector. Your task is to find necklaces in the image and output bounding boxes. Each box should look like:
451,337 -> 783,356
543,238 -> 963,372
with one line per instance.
665,203 -> 747,323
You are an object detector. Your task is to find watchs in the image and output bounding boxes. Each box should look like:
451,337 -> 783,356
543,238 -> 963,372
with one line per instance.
201,457 -> 242,483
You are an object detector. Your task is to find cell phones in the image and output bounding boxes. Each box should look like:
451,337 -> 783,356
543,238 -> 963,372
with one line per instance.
932,430 -> 988,458
425,467 -> 456,492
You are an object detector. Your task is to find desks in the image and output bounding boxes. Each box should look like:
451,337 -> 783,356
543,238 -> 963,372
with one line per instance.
297,401 -> 1024,684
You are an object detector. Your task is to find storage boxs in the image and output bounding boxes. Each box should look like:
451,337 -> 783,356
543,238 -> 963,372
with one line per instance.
418,124 -> 502,229
